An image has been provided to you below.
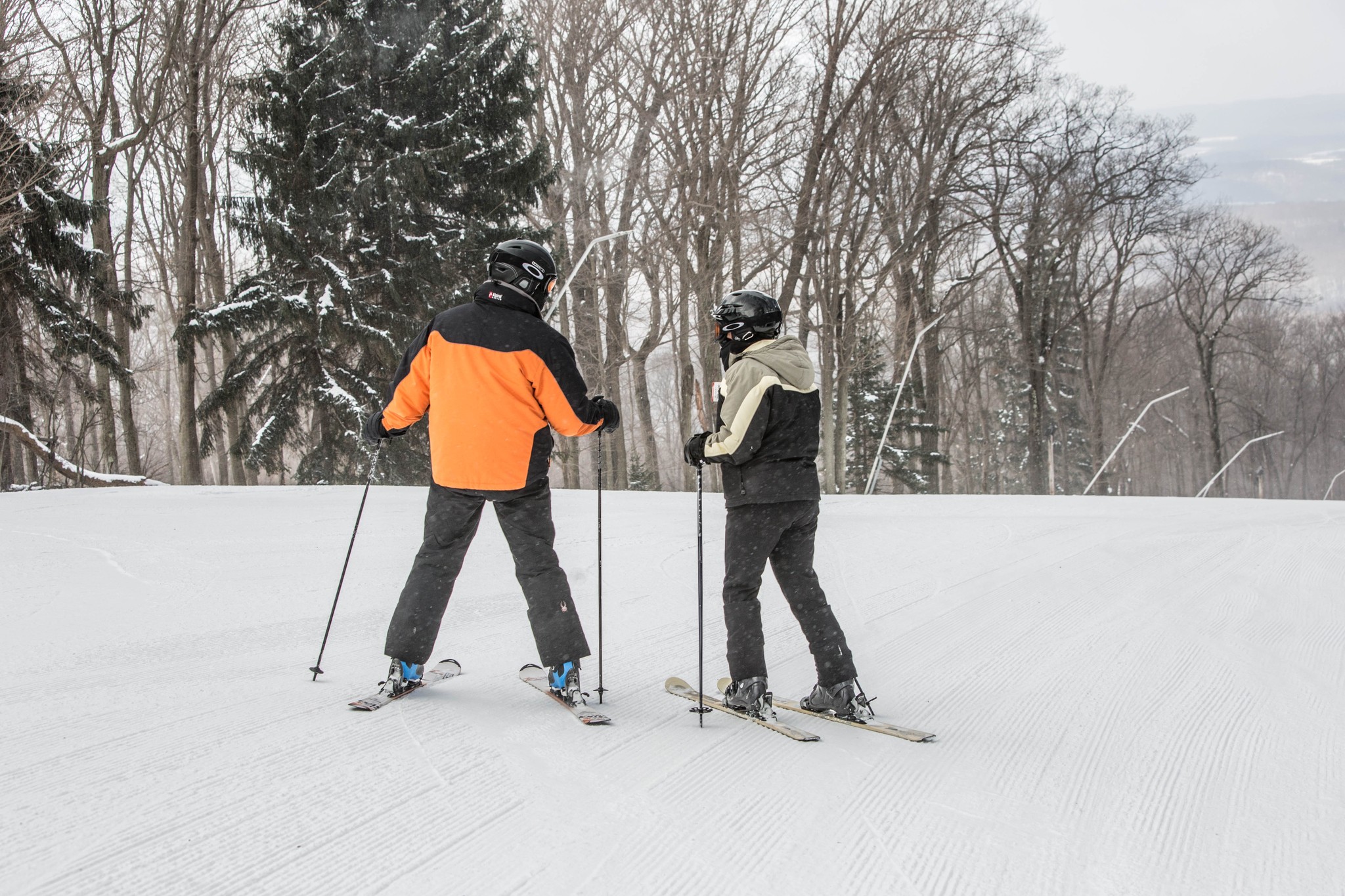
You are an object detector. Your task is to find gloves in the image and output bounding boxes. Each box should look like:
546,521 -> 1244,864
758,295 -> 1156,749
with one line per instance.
363,412 -> 390,447
598,398 -> 620,434
684,431 -> 713,466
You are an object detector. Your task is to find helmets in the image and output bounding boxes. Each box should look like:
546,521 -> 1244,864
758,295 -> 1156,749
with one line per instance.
710,290 -> 782,348
487,240 -> 557,312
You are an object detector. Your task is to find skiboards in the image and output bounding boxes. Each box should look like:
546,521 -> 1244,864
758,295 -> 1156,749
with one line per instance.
663,675 -> 937,748
343,657 -> 613,728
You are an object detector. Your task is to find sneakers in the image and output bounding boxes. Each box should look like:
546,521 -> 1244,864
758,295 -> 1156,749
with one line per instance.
378,658 -> 424,698
548,661 -> 590,708
800,678 -> 876,725
722,676 -> 777,721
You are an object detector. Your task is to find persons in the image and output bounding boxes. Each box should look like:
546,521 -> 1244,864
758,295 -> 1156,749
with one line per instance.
682,290 -> 860,716
361,240 -> 592,707
